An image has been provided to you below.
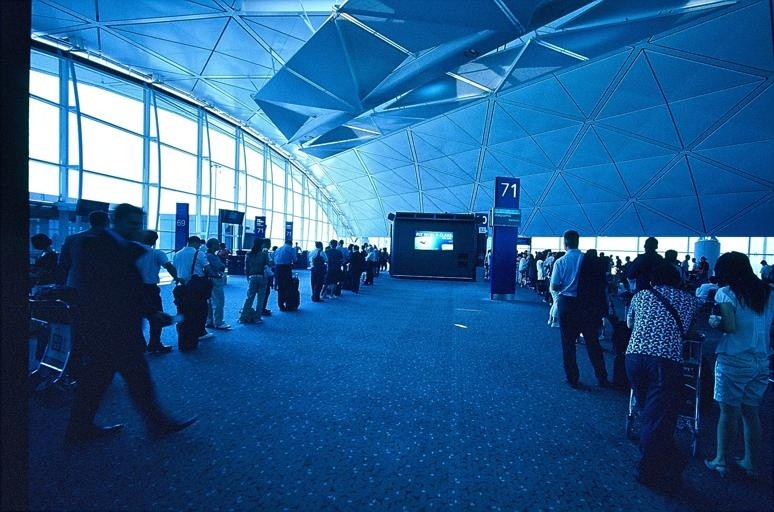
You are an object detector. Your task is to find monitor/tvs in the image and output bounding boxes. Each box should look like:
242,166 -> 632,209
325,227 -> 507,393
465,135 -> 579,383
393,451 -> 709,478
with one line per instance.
413,230 -> 454,251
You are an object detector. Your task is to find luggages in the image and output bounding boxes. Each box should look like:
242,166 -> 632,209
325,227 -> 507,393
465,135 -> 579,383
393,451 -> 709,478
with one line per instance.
292,264 -> 300,309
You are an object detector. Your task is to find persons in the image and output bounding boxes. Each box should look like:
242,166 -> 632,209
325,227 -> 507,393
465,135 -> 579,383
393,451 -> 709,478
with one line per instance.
702,250 -> 774,481
309,240 -> 389,302
551,229 -> 611,388
29,201 -> 202,445
621,263 -> 695,484
515,229 -> 633,307
199,236 -> 303,329
626,229 -> 773,319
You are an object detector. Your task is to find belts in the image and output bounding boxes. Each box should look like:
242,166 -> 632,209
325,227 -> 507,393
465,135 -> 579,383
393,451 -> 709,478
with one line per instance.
249,275 -> 263,278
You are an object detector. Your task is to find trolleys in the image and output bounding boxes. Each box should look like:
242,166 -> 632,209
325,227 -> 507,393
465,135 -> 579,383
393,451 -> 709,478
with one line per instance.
322,262 -> 345,295
28,294 -> 79,394
623,333 -> 707,460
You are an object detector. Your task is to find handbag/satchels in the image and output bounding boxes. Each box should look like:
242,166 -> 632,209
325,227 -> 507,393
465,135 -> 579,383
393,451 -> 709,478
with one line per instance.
263,265 -> 273,278
187,275 -> 212,299
314,256 -> 327,274
239,306 -> 256,322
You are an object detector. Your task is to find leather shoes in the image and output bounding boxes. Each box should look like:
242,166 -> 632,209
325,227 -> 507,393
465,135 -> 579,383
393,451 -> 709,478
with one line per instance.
147,343 -> 172,353
732,455 -> 759,480
83,422 -> 123,437
155,416 -> 200,437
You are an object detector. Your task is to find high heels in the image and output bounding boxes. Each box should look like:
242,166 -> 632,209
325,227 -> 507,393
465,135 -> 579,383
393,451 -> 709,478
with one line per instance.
704,457 -> 730,478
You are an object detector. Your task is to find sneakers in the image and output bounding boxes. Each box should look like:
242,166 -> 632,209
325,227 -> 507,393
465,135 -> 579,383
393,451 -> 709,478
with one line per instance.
196,323 -> 230,339
